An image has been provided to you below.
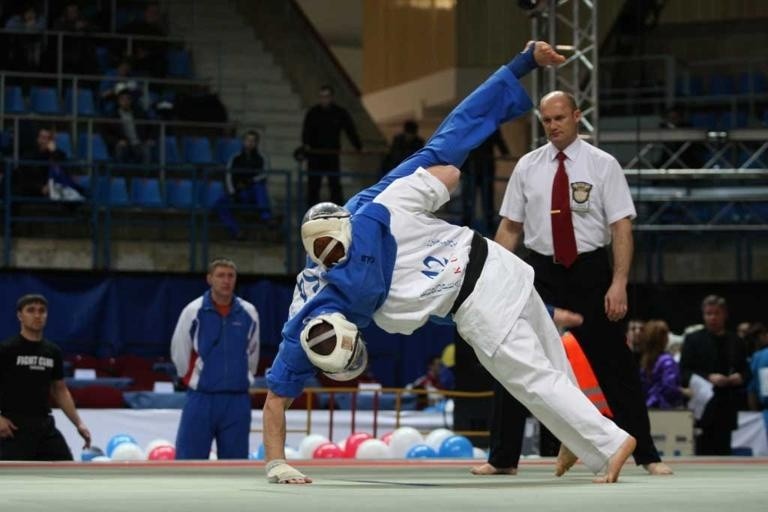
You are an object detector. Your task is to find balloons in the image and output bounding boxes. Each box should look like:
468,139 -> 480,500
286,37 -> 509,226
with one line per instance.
75,422 -> 489,463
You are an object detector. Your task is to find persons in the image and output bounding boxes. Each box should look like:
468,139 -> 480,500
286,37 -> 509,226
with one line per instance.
379,119 -> 428,177
169,255 -> 262,458
0,1 -> 274,243
655,106 -> 696,169
456,127 -> 511,240
1,290 -> 91,460
405,292 -> 767,454
297,196 -> 637,488
261,38 -> 568,488
471,90 -> 680,478
299,83 -> 365,206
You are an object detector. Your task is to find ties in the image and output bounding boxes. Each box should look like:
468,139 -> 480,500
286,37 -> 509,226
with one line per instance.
550,151 -> 579,269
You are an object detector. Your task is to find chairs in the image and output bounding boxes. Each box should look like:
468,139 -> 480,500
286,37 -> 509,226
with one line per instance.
0,81 -> 278,224
603,69 -> 767,227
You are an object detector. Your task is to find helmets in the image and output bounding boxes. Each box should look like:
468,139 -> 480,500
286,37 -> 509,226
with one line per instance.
299,201 -> 355,272
300,309 -> 369,383
